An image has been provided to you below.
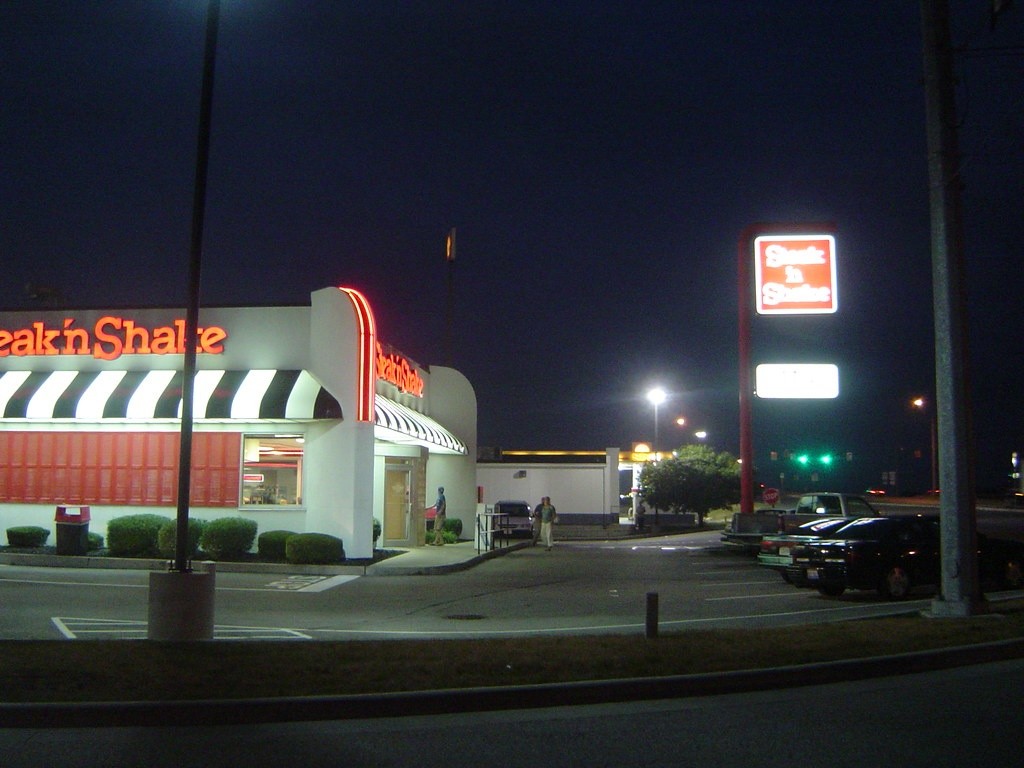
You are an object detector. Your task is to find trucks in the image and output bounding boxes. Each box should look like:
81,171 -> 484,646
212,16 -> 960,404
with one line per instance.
722,490 -> 882,558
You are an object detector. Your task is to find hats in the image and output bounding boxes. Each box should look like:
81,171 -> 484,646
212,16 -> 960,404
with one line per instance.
438,486 -> 444,493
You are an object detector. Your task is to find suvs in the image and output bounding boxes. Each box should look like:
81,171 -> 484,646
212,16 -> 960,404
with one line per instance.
493,500 -> 535,537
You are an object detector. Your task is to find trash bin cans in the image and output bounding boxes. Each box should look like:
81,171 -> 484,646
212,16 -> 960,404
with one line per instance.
54,505 -> 90,556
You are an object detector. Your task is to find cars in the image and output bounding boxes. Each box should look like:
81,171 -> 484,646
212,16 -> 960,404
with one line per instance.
756,516 -> 859,596
795,514 -> 1024,601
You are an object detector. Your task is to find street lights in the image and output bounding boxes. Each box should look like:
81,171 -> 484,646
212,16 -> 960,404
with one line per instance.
913,397 -> 938,493
649,390 -> 666,530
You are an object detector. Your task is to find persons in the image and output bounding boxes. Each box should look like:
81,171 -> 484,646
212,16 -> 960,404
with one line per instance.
424,486 -> 446,545
532,496 -> 555,547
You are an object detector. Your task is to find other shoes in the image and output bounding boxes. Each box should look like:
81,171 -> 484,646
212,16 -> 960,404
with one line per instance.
430,544 -> 436,546
436,544 -> 443,546
545,546 -> 553,551
